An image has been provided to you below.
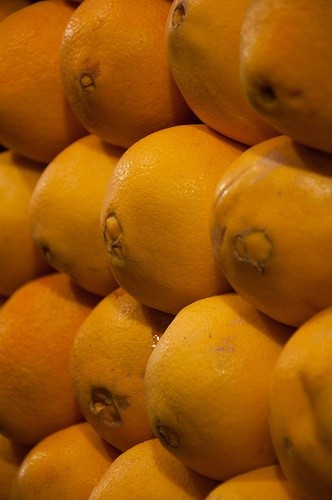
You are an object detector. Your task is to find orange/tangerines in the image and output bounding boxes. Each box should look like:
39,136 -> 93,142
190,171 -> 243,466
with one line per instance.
0,0 -> 332,500
